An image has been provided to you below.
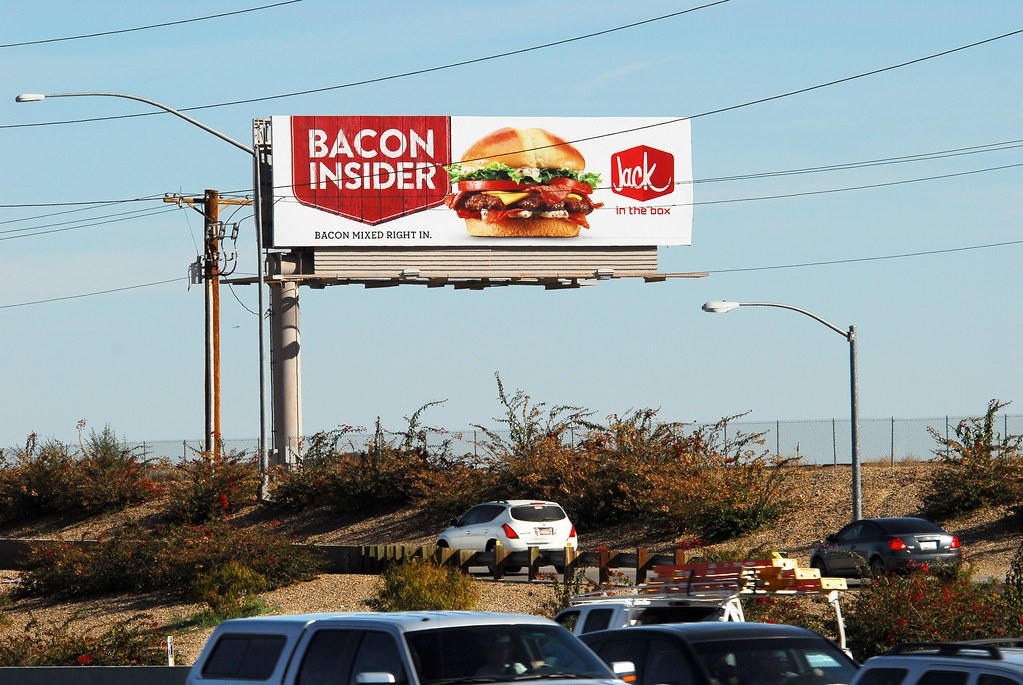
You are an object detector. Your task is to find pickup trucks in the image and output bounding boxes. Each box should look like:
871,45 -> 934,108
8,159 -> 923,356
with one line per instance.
540,590 -> 856,669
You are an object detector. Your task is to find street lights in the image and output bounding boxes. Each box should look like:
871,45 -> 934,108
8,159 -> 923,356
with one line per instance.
15,88 -> 272,501
700,298 -> 863,534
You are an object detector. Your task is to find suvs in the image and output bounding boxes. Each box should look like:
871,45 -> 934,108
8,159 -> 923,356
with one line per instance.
436,498 -> 578,577
849,634 -> 1023,685
540,617 -> 863,685
185,611 -> 638,685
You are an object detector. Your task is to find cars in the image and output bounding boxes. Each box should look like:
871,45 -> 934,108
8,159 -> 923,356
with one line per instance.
808,517 -> 962,586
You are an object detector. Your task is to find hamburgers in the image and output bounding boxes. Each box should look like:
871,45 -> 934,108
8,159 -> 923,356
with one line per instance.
443,127 -> 604,237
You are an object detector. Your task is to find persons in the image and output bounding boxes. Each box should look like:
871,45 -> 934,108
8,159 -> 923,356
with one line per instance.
473,640 -> 546,676
753,652 -> 823,685
